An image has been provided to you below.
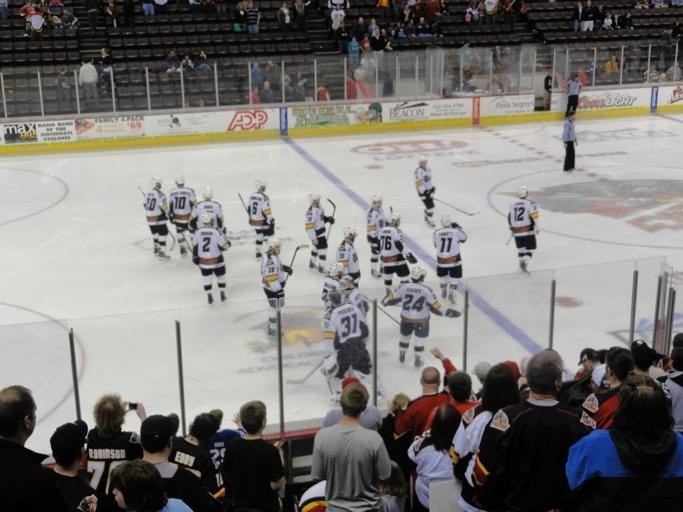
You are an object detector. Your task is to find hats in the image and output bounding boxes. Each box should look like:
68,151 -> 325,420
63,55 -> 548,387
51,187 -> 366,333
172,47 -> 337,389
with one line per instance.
142,412 -> 178,440
629,338 -> 655,369
577,348 -> 596,366
341,375 -> 369,411
471,356 -> 534,381
189,409 -> 222,435
51,418 -> 88,450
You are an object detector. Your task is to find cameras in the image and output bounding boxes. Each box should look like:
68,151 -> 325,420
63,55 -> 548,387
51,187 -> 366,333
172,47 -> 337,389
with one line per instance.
128,401 -> 138,415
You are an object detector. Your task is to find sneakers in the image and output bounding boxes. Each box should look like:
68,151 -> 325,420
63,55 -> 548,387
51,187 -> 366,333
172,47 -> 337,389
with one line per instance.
371,265 -> 385,276
520,260 -> 531,273
441,288 -> 457,303
397,350 -> 407,362
255,251 -> 262,258
309,259 -> 329,274
154,246 -> 199,257
207,294 -> 217,305
220,290 -> 229,301
268,322 -> 283,337
425,210 -> 436,227
414,354 -> 426,368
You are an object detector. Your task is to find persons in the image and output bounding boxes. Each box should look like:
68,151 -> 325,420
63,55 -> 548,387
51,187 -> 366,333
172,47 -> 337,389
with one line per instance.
508,186 -> 541,277
246,180 -> 294,338
431,213 -> 467,306
2,333 -> 682,512
561,111 -> 578,171
1,1 -> 682,121
413,156 -> 438,226
305,194 -> 445,401
141,180 -> 230,305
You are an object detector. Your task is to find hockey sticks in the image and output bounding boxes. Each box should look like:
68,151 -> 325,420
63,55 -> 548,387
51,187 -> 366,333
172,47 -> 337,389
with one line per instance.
326,198 -> 336,241
138,185 -> 176,251
434,198 -> 481,216
285,244 -> 309,281
286,352 -> 331,384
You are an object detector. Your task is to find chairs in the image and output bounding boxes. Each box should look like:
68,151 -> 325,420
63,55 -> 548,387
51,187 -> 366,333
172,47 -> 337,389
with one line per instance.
279,434 -> 323,512
0,0 -> 683,120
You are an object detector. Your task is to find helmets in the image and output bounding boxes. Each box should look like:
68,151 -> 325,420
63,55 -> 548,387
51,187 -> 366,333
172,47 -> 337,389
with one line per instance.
342,224 -> 359,237
440,213 -> 454,226
150,176 -> 215,225
384,211 -> 401,225
321,358 -> 340,377
254,179 -> 267,191
308,191 -> 321,204
517,185 -> 529,199
409,263 -> 427,281
330,262 -> 355,289
265,235 -> 282,248
369,194 -> 381,206
418,155 -> 428,165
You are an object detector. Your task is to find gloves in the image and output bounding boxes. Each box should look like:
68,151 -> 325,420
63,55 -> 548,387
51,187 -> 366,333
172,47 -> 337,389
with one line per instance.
451,221 -> 460,230
282,265 -> 293,275
405,253 -> 417,265
323,215 -> 335,225
445,309 -> 462,319
279,281 -> 286,289
270,218 -> 275,228
